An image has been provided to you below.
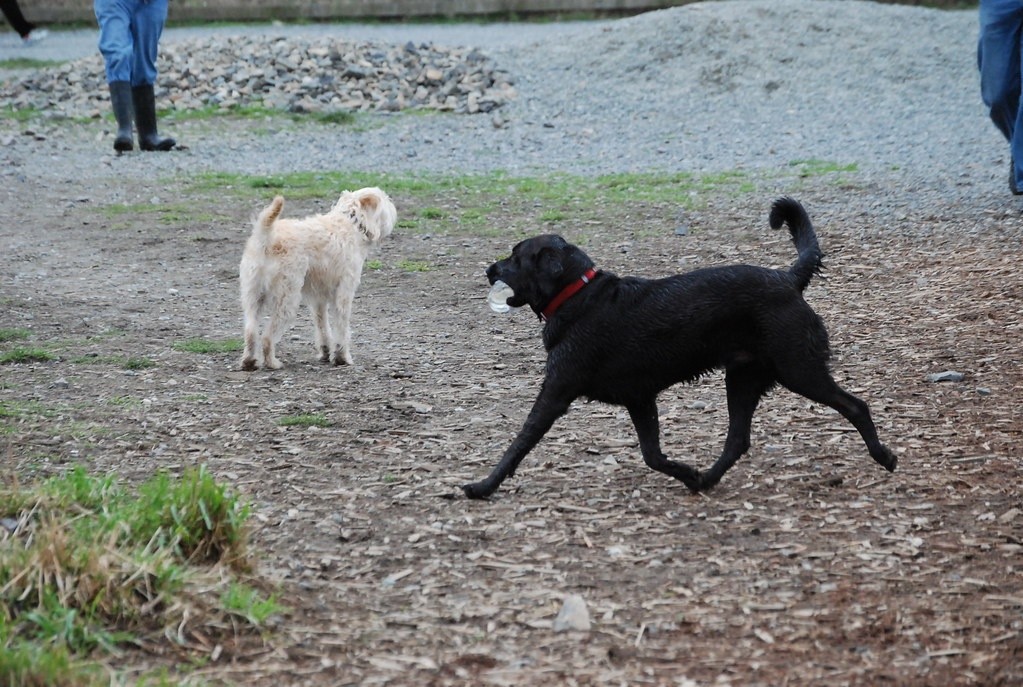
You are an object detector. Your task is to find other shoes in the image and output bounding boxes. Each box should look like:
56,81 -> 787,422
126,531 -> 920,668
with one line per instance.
23,30 -> 48,47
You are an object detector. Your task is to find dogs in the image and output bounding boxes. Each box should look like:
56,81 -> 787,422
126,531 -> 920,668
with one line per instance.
459,195 -> 900,501
237,186 -> 398,373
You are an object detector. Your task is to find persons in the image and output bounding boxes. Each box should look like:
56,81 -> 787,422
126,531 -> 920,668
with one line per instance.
94,0 -> 176,150
977,0 -> 1023,194
0,0 -> 48,49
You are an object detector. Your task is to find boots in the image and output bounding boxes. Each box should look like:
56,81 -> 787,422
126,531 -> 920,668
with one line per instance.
132,84 -> 177,150
109,80 -> 133,150
